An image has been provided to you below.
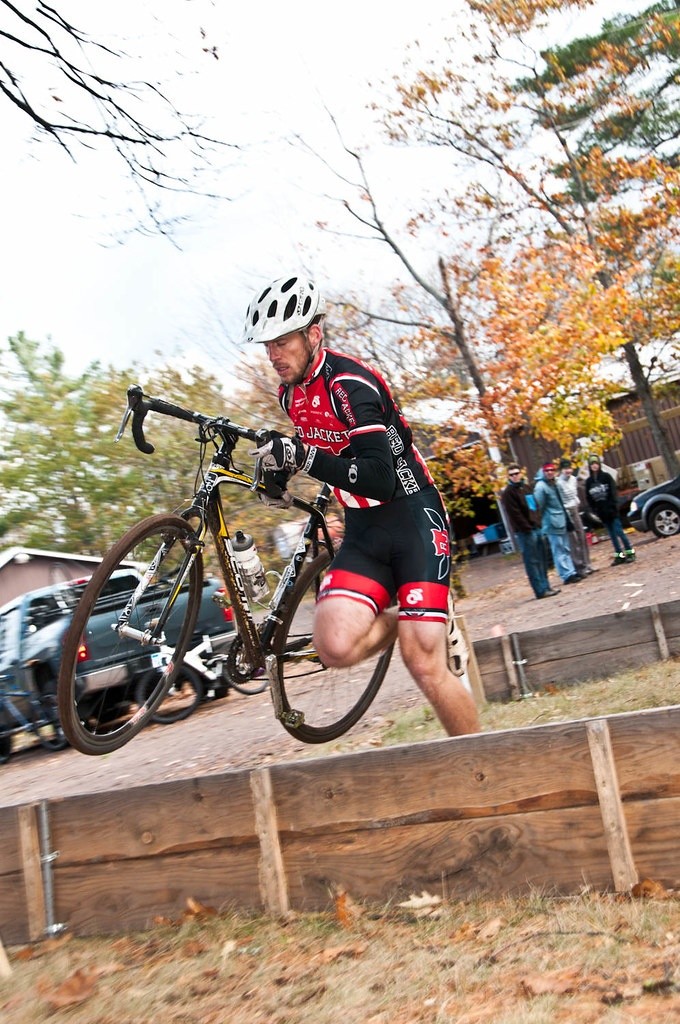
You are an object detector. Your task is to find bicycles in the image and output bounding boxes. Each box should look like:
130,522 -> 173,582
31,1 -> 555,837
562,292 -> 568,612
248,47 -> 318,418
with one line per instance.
57,383 -> 398,758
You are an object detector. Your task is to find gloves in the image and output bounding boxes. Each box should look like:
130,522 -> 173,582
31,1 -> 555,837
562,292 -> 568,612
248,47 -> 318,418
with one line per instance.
247,430 -> 305,472
259,467 -> 298,509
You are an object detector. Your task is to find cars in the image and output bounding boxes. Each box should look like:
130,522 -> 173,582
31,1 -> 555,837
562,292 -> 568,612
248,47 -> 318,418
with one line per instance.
625,469 -> 680,539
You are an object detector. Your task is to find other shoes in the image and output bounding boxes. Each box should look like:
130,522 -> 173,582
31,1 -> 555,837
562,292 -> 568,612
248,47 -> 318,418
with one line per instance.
586,567 -> 599,574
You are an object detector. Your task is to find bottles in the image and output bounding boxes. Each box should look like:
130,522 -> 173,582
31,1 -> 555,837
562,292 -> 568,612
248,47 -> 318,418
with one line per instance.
231,529 -> 270,601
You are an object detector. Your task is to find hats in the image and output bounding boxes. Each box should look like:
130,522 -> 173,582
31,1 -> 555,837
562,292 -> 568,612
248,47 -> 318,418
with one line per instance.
590,456 -> 599,464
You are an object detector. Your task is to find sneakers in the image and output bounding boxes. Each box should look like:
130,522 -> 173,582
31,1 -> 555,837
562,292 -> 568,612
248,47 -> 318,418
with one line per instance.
611,557 -> 625,566
564,575 -> 581,584
577,574 -> 587,579
626,553 -> 636,563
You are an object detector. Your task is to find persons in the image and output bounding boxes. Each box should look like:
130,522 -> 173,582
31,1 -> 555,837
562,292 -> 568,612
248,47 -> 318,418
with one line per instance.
244,275 -> 481,741
533,463 -> 587,585
585,455 -> 636,566
556,459 -> 598,576
502,465 -> 561,599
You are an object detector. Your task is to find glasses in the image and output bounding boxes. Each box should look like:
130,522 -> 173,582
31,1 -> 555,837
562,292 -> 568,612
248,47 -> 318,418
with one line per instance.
510,472 -> 520,476
545,469 -> 555,472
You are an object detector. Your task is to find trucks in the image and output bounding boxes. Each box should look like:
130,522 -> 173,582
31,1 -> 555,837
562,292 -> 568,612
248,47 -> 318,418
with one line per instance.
0,566 -> 236,762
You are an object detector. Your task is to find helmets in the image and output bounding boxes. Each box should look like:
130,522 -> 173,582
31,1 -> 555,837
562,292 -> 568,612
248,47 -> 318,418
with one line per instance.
242,274 -> 327,343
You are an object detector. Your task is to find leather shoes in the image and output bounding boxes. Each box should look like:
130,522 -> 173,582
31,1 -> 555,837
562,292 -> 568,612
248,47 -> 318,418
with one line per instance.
537,588 -> 561,599
